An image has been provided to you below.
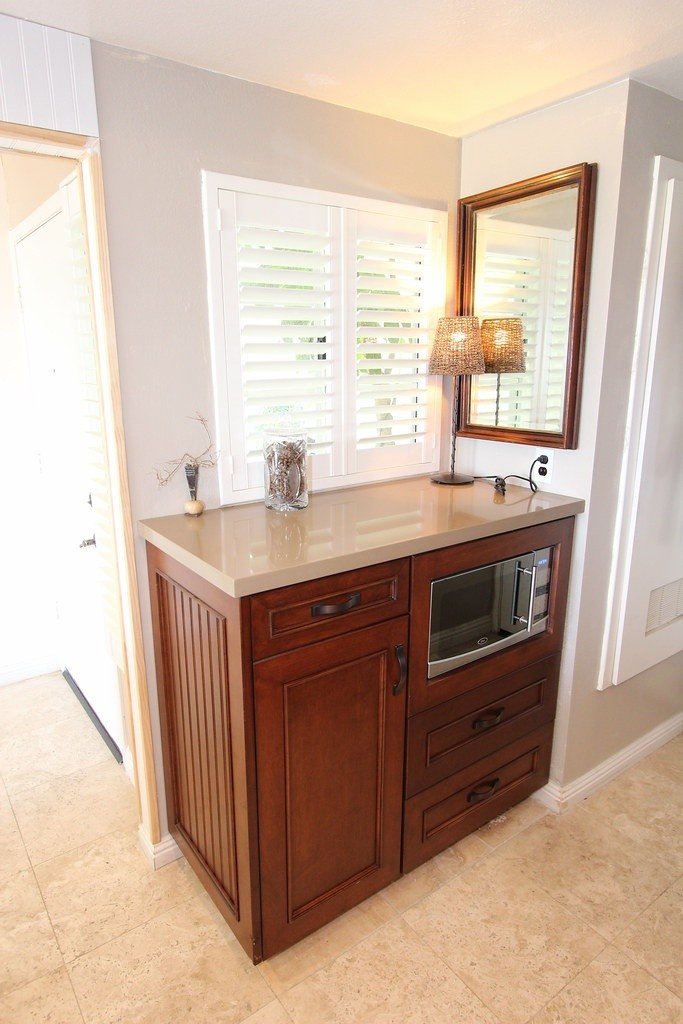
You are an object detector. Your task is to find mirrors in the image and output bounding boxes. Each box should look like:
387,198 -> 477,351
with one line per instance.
454,159 -> 588,451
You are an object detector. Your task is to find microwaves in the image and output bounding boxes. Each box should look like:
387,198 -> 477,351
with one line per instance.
428,545 -> 554,677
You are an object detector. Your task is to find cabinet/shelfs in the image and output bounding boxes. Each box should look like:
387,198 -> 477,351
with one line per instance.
145,516 -> 577,966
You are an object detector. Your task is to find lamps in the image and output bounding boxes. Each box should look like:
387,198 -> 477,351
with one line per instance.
428,317 -> 485,485
481,317 -> 524,426
